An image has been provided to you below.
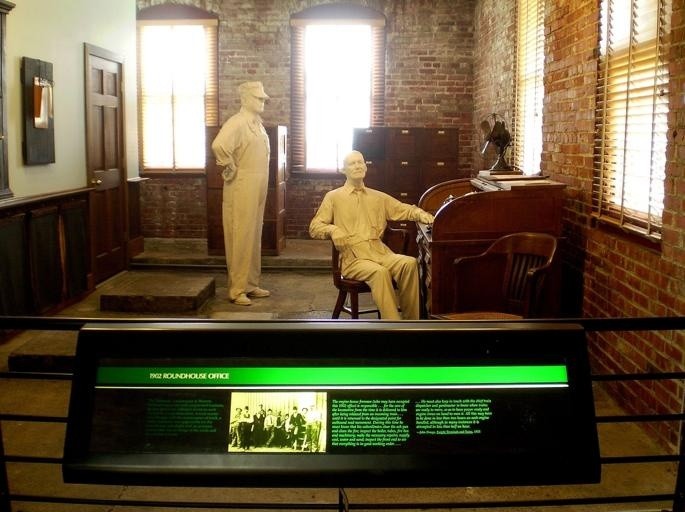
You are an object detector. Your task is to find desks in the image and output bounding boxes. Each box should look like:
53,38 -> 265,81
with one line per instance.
415,177 -> 567,319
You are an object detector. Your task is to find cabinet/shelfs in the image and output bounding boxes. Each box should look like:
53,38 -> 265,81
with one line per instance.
353,126 -> 458,258
204,124 -> 288,257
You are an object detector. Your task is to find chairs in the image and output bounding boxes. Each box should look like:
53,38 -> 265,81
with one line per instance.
332,223 -> 411,319
429,231 -> 559,321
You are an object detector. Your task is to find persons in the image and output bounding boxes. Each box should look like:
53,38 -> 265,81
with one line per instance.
310,149 -> 436,322
212,80 -> 271,306
230,405 -> 323,452
